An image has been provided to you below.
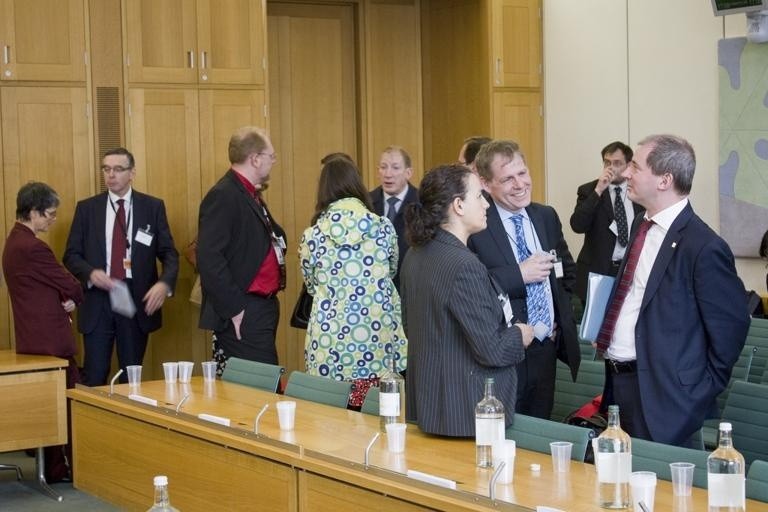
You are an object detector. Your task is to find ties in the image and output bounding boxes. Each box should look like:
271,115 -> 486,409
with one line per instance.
593,221 -> 656,360
387,197 -> 400,223
614,187 -> 628,247
110,199 -> 126,280
256,192 -> 286,289
509,215 -> 551,342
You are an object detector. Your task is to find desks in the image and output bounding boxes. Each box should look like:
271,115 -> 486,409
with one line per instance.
1,350 -> 69,504
60,376 -> 766,511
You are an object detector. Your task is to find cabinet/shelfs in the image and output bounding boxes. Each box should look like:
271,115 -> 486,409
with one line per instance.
121,0 -> 267,86
0,82 -> 96,380
427,92 -> 546,205
125,83 -> 281,383
427,0 -> 543,89
1,0 -> 89,83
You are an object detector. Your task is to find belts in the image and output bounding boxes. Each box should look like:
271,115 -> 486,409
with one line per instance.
604,359 -> 637,374
248,290 -> 277,300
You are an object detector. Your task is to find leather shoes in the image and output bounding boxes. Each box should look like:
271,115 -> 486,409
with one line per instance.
47,469 -> 72,483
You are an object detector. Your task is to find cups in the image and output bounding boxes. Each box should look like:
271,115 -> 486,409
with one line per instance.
178,383 -> 195,402
551,473 -> 573,501
669,461 -> 695,497
384,421 -> 407,453
279,430 -> 296,444
129,385 -> 142,399
163,362 -> 178,382
203,383 -> 217,403
491,439 -> 515,483
179,361 -> 195,382
277,400 -> 296,429
672,498 -> 693,512
384,452 -> 406,472
535,249 -> 553,279
632,470 -> 658,511
202,361 -> 215,383
550,441 -> 575,474
125,363 -> 143,385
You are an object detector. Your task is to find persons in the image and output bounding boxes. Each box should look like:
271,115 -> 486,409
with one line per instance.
567,142 -> 647,363
458,136 -> 490,167
181,237 -> 229,382
196,127 -> 290,395
61,148 -> 180,389
0,181 -> 87,487
298,149 -> 408,384
366,146 -> 421,297
595,134 -> 750,451
396,164 -> 533,439
465,139 -> 586,421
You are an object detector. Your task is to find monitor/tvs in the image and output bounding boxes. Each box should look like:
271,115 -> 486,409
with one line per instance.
711,0 -> 768,16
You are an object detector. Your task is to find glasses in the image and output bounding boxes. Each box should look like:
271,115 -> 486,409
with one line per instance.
102,165 -> 130,172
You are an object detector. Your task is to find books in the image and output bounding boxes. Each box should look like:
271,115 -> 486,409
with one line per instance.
106,278 -> 137,319
576,271 -> 616,341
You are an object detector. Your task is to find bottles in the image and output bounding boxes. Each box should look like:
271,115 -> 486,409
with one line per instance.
595,404 -> 632,508
707,421 -> 746,511
379,350 -> 405,433
164,382 -> 178,403
145,475 -> 177,512
475,374 -> 506,466
